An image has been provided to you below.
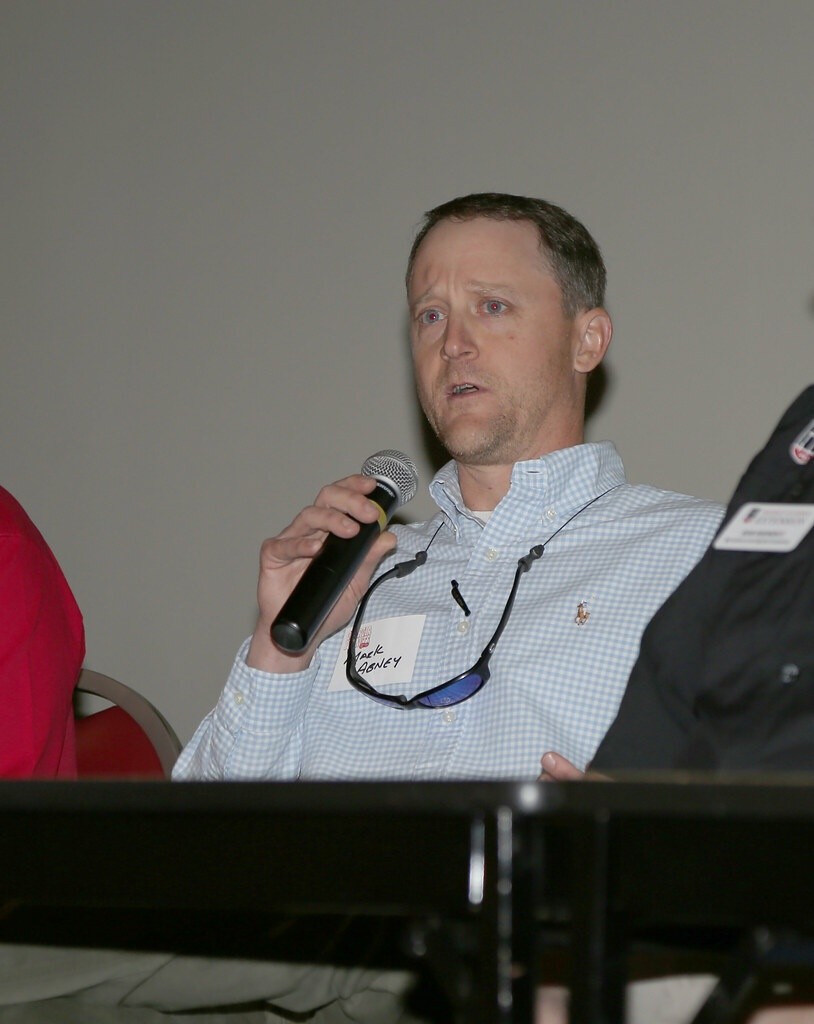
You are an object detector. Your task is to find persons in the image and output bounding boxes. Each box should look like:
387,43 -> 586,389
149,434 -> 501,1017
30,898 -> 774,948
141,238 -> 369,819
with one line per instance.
0,483 -> 87,782
0,194 -> 726,1024
397,383 -> 814,1024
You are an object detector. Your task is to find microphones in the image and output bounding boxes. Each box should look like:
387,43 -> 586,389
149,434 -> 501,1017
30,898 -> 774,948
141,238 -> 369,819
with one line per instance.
272,450 -> 420,652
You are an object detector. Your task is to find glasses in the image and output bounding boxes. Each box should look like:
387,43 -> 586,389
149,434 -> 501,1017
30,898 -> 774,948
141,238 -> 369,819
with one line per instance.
347,560 -> 523,710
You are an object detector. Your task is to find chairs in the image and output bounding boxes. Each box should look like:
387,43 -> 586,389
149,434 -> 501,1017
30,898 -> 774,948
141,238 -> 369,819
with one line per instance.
72,668 -> 182,779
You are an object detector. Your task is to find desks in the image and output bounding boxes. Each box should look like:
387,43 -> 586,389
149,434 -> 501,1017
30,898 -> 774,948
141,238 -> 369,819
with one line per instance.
0,773 -> 814,1024
